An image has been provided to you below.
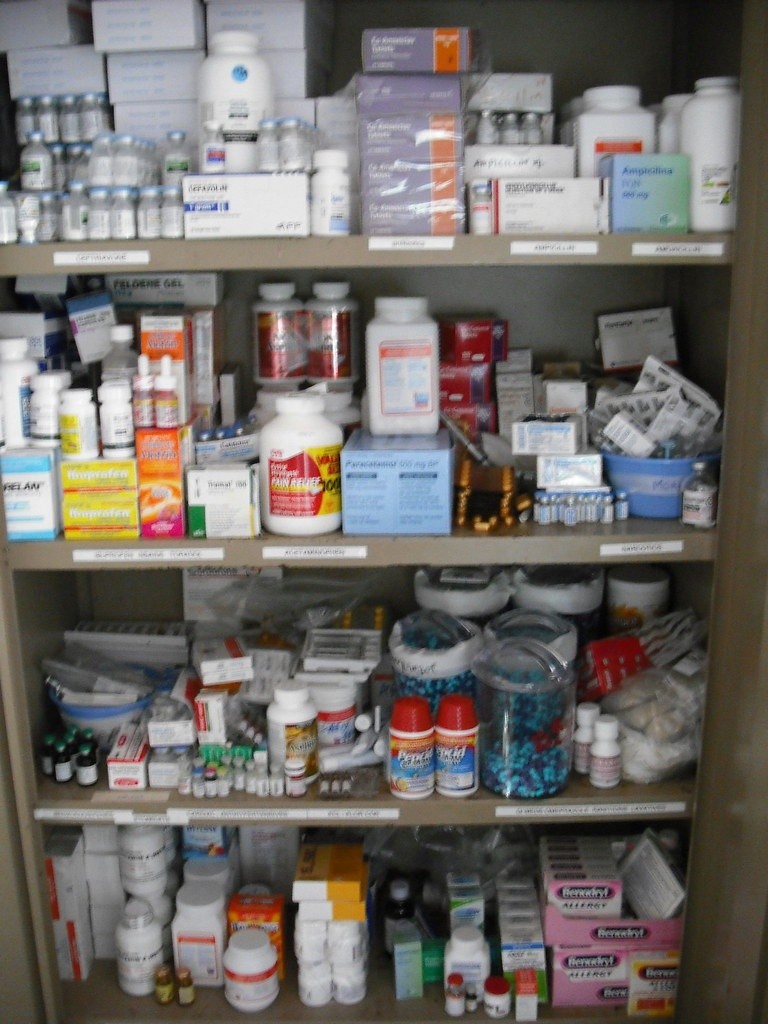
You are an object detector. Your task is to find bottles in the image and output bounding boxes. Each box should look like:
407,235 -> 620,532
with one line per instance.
0,28 -> 747,1024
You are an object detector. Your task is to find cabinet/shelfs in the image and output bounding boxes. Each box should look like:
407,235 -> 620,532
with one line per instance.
1,1 -> 766,1024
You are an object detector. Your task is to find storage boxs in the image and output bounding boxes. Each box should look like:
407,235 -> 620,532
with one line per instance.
341,307 -> 678,536
0,272 -> 261,542
292,844 -> 373,923
0,0 -> 692,238
227,894 -> 285,983
43,826 -> 125,981
446,837 -> 684,1018
106,637 -> 254,789
